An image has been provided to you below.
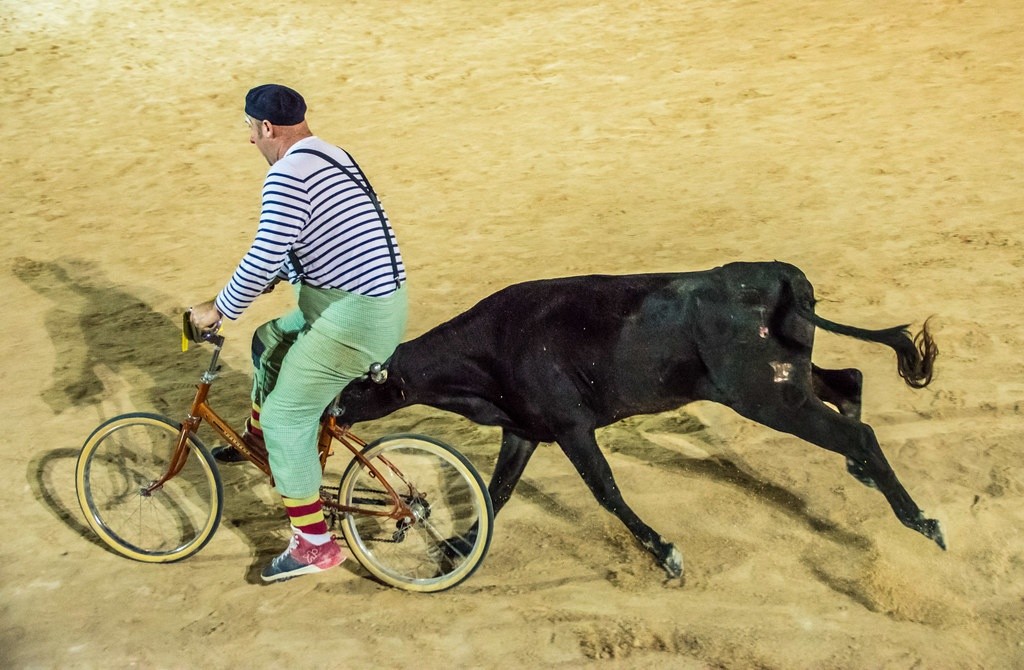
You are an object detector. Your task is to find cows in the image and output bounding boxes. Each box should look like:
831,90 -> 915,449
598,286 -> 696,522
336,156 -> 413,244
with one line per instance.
324,259 -> 947,591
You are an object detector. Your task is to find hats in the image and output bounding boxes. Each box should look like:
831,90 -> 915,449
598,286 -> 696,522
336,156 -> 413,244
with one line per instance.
245,84 -> 306,126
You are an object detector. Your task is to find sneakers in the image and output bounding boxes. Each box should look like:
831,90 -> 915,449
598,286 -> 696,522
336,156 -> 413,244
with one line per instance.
261,523 -> 347,581
211,417 -> 267,466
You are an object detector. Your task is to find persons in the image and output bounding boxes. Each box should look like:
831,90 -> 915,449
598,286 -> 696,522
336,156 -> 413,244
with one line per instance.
184,83 -> 405,581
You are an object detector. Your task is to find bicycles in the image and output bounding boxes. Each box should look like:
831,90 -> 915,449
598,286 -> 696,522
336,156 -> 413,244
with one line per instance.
75,280 -> 495,593
